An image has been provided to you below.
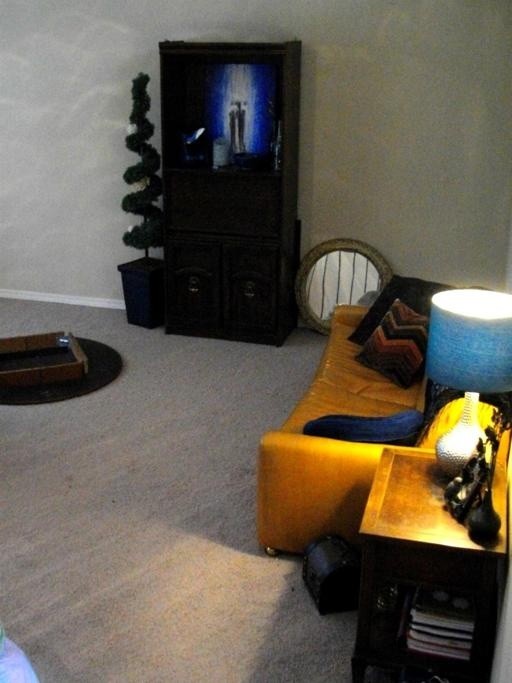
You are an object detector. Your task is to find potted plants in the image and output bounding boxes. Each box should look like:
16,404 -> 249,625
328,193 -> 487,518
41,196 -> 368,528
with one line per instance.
113,73 -> 171,328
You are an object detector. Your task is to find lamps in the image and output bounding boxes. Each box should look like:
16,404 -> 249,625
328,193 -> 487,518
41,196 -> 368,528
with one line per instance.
428,286 -> 512,474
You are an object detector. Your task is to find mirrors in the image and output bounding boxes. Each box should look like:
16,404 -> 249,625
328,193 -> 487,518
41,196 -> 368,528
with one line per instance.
292,237 -> 393,338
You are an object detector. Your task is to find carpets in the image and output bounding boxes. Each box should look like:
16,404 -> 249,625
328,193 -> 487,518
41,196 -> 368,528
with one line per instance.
0,333 -> 122,405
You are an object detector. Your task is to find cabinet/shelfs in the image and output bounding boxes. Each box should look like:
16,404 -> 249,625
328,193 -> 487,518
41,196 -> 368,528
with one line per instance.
156,41 -> 302,347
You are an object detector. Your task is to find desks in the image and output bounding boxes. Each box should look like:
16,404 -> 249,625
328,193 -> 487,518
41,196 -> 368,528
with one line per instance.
351,447 -> 507,680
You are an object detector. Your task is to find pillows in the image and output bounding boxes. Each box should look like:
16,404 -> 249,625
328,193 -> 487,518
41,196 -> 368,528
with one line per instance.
303,407 -> 425,445
348,274 -> 452,390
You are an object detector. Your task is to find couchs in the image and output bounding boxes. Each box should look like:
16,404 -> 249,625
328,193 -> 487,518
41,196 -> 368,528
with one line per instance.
254,305 -> 509,562
